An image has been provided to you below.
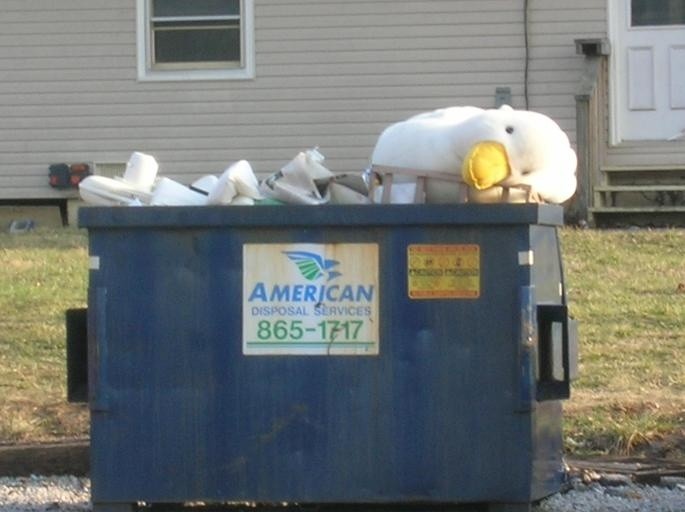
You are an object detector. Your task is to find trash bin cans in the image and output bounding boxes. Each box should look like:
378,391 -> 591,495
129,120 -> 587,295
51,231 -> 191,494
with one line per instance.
64,206 -> 579,510
